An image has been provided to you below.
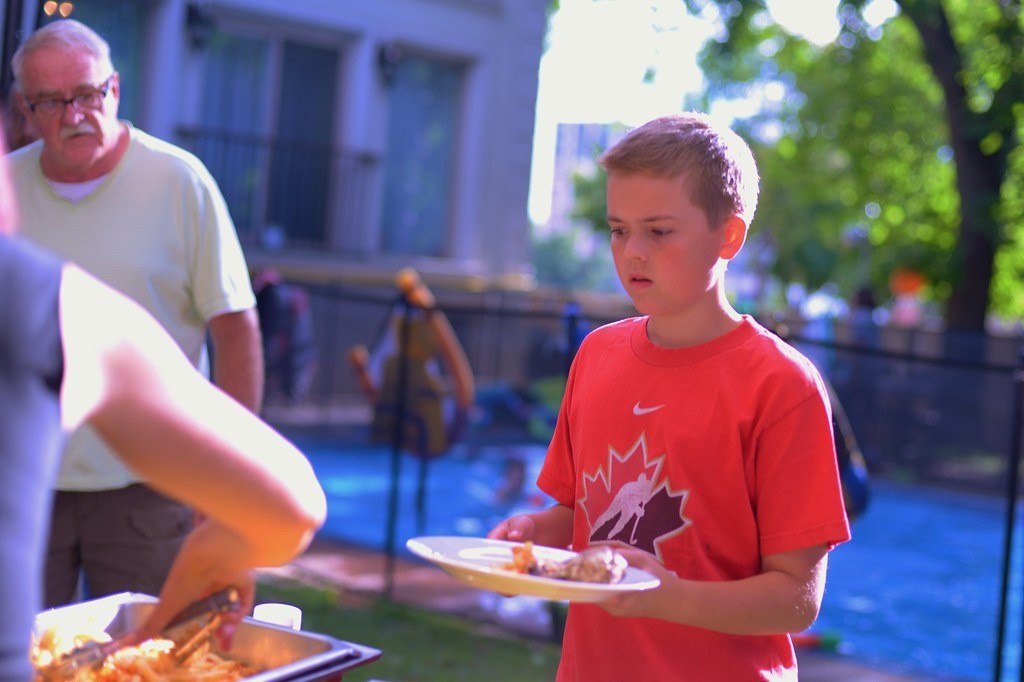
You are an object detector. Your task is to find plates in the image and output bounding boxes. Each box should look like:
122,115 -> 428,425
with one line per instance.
406,535 -> 660,603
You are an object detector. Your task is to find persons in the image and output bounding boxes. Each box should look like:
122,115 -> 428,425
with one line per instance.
0,228 -> 328,682
484,111 -> 851,682
0,18 -> 265,609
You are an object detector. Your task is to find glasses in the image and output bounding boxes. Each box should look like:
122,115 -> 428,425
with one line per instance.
23,79 -> 110,118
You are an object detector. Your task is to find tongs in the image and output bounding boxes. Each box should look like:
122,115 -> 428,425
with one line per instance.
34,586 -> 241,682
